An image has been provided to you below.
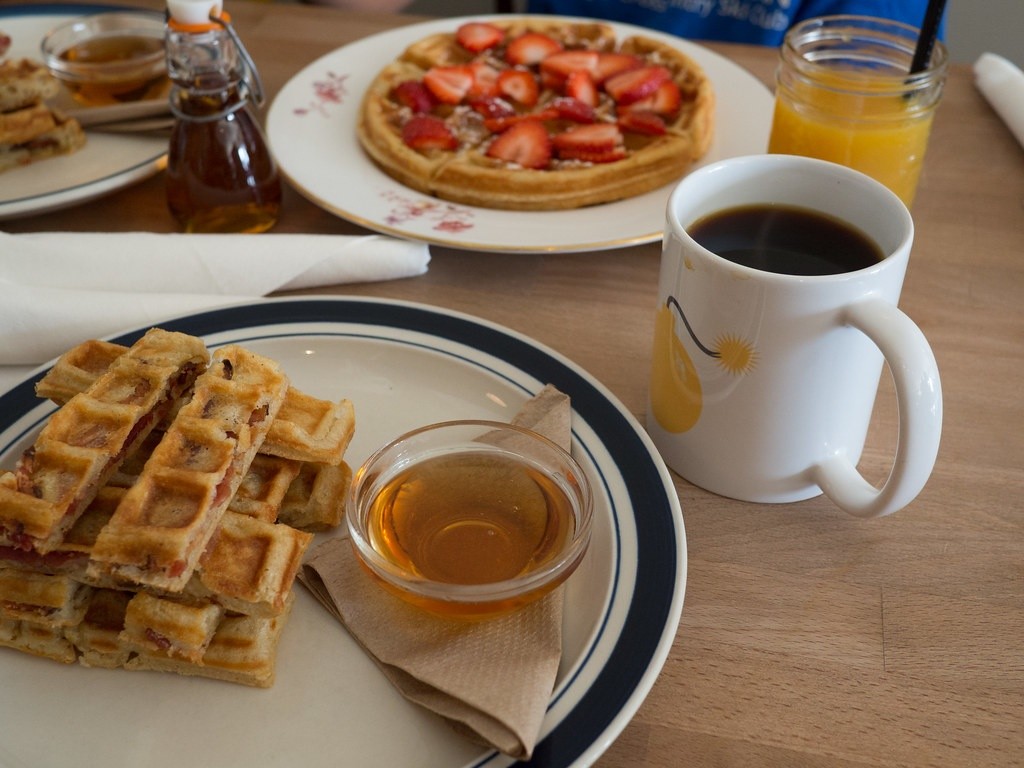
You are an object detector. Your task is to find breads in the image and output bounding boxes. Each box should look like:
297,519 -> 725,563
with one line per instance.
1,327 -> 357,689
0,56 -> 86,175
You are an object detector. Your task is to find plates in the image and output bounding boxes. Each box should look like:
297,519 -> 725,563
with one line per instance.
0,13 -> 169,218
266,14 -> 777,253
0,295 -> 687,768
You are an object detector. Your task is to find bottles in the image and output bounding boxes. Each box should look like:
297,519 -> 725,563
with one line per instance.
166,0 -> 280,233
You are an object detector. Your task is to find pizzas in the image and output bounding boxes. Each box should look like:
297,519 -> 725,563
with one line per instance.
356,16 -> 714,210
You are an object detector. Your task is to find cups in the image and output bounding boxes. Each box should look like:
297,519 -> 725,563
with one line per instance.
647,155 -> 942,520
767,15 -> 950,211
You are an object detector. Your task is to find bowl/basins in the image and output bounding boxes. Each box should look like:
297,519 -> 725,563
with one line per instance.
40,16 -> 168,105
344,420 -> 593,624
